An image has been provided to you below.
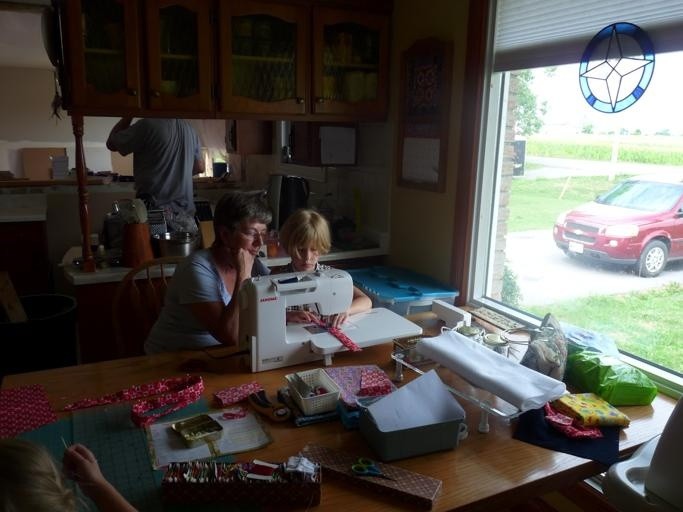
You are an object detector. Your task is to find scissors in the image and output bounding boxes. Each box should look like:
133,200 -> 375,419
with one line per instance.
351,457 -> 397,481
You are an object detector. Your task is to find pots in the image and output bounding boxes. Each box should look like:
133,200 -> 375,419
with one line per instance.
152,230 -> 200,264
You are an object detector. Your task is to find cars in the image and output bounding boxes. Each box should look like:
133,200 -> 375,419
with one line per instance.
552,176 -> 682,277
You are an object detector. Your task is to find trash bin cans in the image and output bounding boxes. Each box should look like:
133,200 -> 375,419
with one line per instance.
0,294 -> 80,377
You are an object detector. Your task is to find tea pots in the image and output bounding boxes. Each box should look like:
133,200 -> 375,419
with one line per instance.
121,223 -> 154,269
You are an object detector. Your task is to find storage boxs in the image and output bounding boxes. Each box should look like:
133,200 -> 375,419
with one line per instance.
348,264 -> 460,316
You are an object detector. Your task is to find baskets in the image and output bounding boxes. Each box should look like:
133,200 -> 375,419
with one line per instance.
284,368 -> 342,416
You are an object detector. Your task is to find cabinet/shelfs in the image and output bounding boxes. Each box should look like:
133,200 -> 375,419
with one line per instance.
288,121 -> 359,167
224,119 -> 272,155
215,0 -> 393,120
60,1 -> 216,117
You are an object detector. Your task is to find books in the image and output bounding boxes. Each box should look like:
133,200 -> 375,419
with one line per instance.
52,156 -> 69,179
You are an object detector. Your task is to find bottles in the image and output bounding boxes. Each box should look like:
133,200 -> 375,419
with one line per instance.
482,333 -> 508,360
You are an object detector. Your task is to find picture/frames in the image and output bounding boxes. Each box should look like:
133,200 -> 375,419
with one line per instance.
395,37 -> 454,194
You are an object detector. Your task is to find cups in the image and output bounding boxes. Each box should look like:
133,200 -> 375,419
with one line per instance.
212,163 -> 226,176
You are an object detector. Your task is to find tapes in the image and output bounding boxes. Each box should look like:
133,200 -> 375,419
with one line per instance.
457,423 -> 469,441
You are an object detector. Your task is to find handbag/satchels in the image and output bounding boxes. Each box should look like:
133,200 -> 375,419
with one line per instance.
500,312 -> 567,381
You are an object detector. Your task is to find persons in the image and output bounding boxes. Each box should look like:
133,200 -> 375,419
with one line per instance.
0,437 -> 138,512
273,208 -> 372,329
106,117 -> 205,258
143,190 -> 273,355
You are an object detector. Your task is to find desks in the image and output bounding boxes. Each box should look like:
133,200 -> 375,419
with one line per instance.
0,305 -> 679,510
61,236 -> 390,366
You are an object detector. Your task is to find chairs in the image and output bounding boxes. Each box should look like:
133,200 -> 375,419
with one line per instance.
111,255 -> 187,358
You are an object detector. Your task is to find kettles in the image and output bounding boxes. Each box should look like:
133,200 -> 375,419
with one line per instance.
279,177 -> 311,230
